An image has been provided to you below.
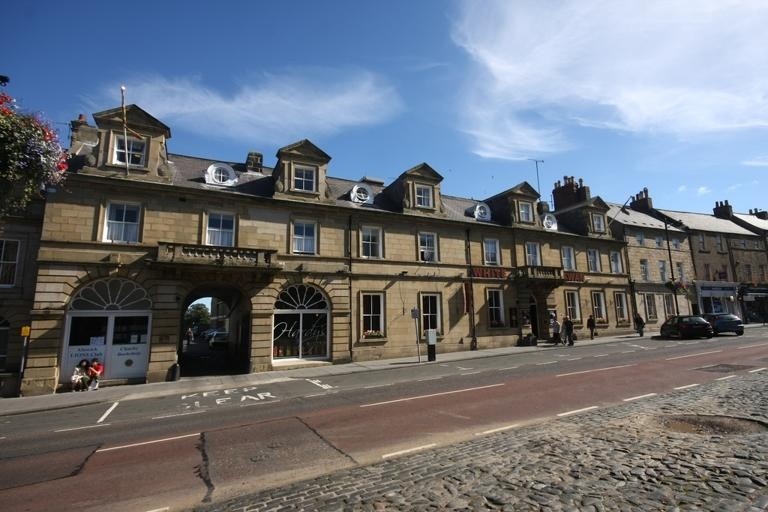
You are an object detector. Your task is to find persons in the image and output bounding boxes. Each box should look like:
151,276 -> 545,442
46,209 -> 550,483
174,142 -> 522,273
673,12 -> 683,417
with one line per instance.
565,316 -> 574,346
587,315 -> 595,340
560,316 -> 567,343
550,318 -> 563,345
86,358 -> 104,390
635,313 -> 644,336
71,359 -> 90,392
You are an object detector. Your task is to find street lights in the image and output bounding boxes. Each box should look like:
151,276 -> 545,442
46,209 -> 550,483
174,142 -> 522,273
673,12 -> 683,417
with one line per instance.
663,216 -> 682,317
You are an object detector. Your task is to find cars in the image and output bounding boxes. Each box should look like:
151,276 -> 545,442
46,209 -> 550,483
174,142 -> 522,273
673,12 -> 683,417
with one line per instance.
659,314 -> 714,339
199,328 -> 229,351
699,312 -> 744,336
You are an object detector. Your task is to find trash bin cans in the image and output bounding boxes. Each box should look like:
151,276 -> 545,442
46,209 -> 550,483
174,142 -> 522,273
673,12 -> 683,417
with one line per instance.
0,372 -> 18,397
